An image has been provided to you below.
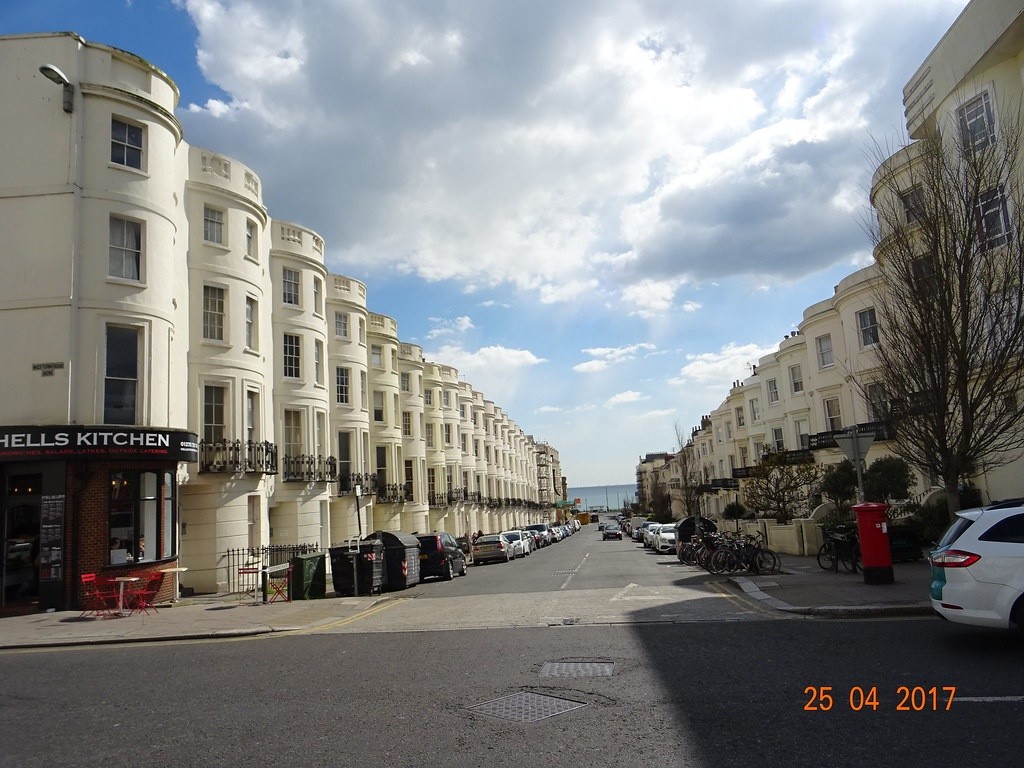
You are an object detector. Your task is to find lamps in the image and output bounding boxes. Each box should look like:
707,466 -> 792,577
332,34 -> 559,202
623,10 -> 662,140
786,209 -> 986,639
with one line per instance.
38,62 -> 75,113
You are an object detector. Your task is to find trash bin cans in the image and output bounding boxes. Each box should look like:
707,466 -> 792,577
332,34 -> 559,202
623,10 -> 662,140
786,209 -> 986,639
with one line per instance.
673,515 -> 718,558
292,552 -> 326,600
363,529 -> 421,590
328,539 -> 383,597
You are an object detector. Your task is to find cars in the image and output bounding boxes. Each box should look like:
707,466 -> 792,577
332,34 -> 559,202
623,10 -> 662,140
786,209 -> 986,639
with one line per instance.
473,518 -> 582,565
409,530 -> 467,582
927,497 -> 1024,637
602,523 -> 623,541
597,522 -> 609,531
616,513 -> 677,554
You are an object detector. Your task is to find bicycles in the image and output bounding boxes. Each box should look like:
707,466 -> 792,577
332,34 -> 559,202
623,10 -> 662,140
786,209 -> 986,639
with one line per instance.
815,524 -> 859,573
678,524 -> 782,576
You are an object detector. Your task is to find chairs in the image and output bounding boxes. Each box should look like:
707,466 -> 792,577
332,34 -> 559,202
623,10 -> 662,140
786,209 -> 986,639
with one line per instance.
124,569 -> 162,620
80,572 -> 124,619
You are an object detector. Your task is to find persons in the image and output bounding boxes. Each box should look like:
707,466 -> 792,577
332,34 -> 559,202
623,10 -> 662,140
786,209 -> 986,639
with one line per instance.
464,530 -> 484,540
109,537 -> 121,550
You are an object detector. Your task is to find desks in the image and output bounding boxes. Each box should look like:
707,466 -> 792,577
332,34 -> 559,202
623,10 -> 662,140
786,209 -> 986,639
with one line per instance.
94,575 -> 139,617
